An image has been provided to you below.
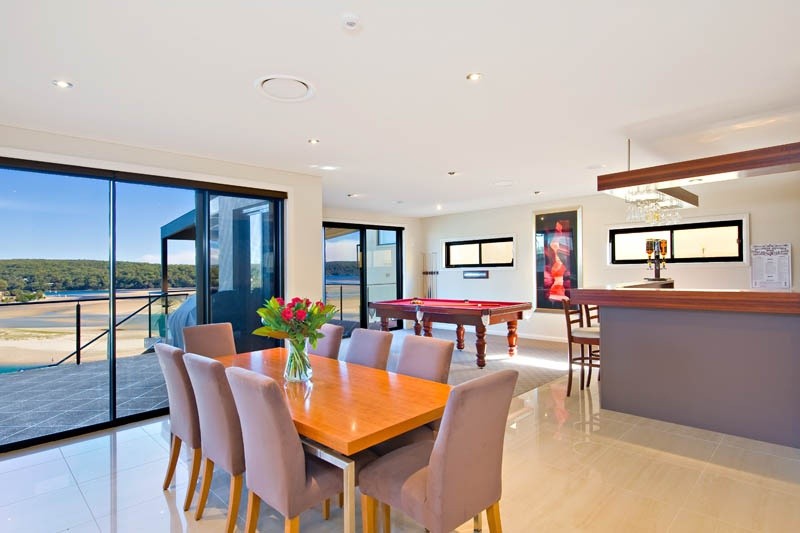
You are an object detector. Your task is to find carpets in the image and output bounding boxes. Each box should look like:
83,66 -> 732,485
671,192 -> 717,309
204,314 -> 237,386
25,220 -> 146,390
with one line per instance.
448,350 -> 569,398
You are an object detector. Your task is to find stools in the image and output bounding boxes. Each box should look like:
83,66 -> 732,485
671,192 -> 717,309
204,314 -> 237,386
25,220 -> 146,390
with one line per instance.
561,299 -> 601,397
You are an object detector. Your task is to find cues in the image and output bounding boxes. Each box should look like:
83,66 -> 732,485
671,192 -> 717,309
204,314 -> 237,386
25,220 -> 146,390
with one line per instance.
422,251 -> 438,302
433,304 -> 500,306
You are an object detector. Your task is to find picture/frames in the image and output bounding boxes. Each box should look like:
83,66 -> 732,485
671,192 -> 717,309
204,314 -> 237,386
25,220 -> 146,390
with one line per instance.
532,205 -> 583,314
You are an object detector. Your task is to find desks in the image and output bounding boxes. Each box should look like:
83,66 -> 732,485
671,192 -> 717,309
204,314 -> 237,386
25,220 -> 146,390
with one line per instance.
211,347 -> 482,533
368,297 -> 532,369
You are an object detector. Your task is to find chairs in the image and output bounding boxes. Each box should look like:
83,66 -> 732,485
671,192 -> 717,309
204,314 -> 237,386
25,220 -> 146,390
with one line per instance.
224,367 -> 378,533
307,323 -> 343,359
154,342 -> 202,511
345,328 -> 393,370
359,371 -> 518,533
182,352 -> 245,533
183,322 -> 236,358
367,329 -> 454,456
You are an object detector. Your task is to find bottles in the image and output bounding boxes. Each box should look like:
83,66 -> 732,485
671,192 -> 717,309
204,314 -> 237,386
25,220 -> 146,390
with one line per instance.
646,238 -> 667,269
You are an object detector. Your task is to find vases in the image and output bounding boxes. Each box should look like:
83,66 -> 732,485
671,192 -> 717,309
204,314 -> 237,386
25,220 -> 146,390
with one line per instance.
283,338 -> 313,382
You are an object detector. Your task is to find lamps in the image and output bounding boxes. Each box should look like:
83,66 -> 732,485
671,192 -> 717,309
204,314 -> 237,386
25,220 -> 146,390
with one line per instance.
626,138 -> 681,225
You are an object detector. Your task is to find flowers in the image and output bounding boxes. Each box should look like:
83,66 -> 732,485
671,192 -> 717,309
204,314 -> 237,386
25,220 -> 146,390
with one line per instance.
254,295 -> 340,380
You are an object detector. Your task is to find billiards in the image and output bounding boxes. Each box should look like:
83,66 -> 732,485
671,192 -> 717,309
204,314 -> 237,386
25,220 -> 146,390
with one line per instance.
410,299 -> 424,304
477,304 -> 482,307
